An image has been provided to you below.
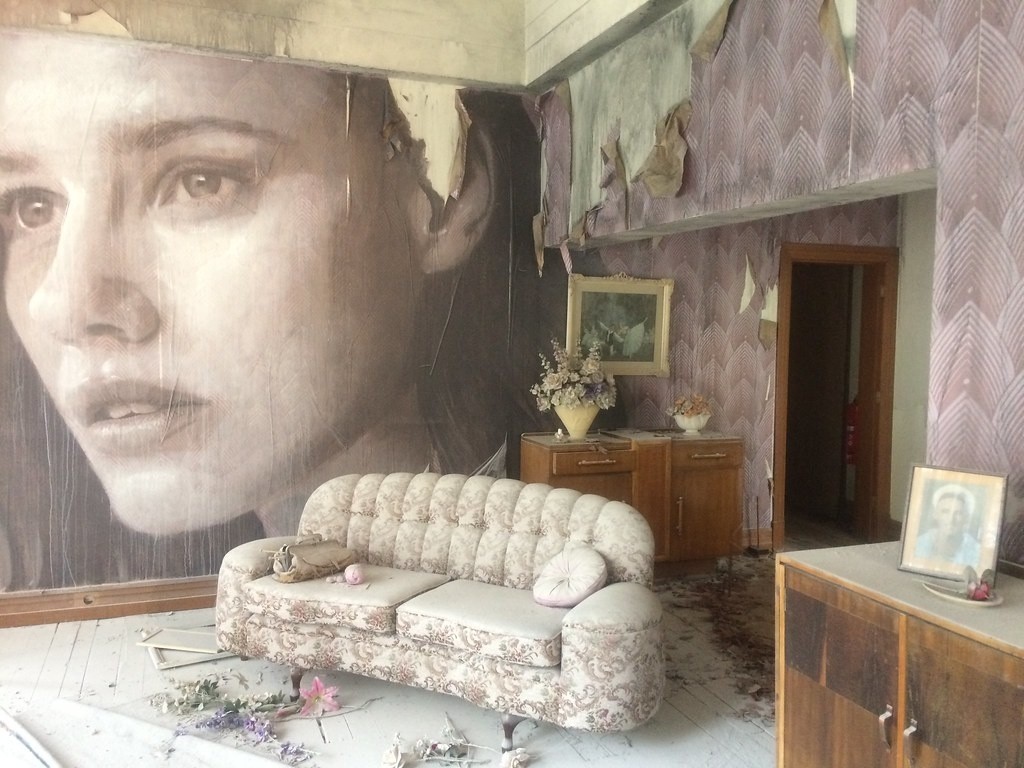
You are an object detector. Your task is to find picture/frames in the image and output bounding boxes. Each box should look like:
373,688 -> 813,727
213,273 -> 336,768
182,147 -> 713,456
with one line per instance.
897,462 -> 1010,589
148,623 -> 238,671
136,627 -> 224,654
566,272 -> 675,378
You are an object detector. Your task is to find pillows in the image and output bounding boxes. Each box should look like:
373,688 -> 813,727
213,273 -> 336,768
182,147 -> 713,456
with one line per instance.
533,546 -> 607,608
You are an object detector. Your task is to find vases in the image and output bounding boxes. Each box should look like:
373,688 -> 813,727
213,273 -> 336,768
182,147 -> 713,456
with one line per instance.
673,414 -> 711,436
550,403 -> 601,441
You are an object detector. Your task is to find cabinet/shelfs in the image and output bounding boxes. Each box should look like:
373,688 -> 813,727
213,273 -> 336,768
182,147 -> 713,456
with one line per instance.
643,428 -> 745,562
774,539 -> 1024,768
598,427 -> 671,562
520,431 -> 637,507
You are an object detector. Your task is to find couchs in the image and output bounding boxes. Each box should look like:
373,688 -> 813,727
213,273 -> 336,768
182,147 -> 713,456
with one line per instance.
215,471 -> 667,757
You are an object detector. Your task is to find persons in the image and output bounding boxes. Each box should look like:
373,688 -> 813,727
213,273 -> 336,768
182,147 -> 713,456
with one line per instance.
0,28 -> 499,542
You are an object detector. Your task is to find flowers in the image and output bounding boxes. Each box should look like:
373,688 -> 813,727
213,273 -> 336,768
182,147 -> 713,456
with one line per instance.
665,393 -> 714,417
146,673 -> 530,768
911,568 -> 997,602
529,329 -> 617,412
